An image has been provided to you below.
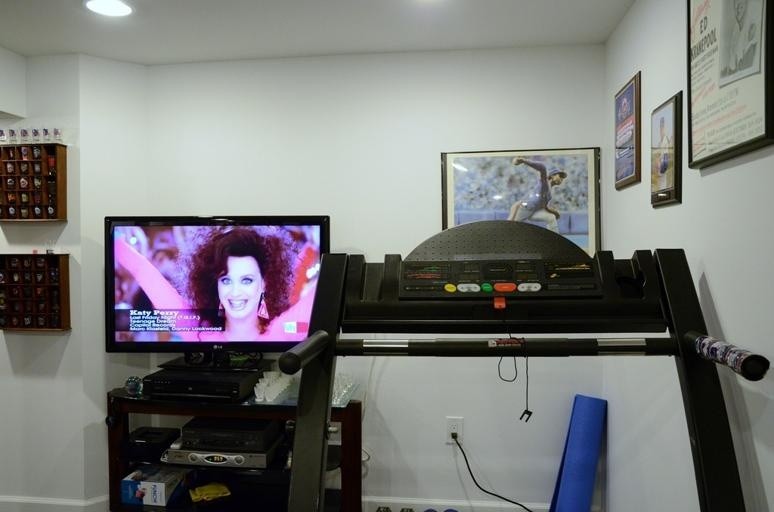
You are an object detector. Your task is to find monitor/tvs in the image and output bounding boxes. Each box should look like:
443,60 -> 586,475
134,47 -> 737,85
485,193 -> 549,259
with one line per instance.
104,215 -> 332,375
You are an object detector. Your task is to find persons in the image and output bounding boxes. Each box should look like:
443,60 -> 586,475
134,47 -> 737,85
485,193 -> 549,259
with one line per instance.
504,157 -> 567,236
657,117 -> 672,190
725,0 -> 758,78
114,227 -> 320,340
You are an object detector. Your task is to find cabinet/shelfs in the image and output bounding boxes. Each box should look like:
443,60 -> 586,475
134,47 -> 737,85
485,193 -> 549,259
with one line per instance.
0,144 -> 59,329
106,384 -> 365,512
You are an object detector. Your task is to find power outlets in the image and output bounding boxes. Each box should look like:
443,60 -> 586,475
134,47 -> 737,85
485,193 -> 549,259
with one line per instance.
446,416 -> 465,446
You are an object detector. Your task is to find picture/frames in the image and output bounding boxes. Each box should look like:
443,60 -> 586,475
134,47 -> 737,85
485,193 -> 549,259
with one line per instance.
686,0 -> 772,169
613,73 -> 640,191
649,89 -> 683,210
439,146 -> 603,262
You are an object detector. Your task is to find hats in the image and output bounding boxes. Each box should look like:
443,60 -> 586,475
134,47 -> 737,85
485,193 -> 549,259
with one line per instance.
547,166 -> 567,180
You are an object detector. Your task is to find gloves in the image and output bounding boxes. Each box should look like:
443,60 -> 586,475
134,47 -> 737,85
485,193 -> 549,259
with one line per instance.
549,208 -> 560,220
512,157 -> 524,166
